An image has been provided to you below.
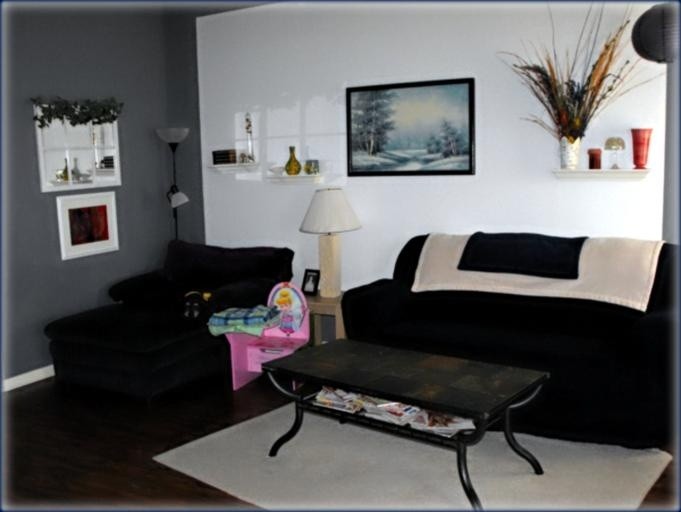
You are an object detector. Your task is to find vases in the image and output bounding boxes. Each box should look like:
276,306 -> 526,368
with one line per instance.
561,137 -> 580,169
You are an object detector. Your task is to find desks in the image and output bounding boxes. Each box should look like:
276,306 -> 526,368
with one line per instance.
305,290 -> 346,344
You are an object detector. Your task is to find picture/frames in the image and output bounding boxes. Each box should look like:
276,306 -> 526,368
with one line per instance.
301,269 -> 320,296
56,191 -> 119,261
346,77 -> 475,175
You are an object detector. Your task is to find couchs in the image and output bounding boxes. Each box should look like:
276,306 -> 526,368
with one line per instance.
342,232 -> 677,451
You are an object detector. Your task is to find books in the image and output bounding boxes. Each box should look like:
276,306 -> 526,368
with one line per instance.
313,384 -> 479,439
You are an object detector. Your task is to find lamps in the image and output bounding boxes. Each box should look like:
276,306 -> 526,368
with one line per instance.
156,128 -> 189,242
299,188 -> 362,298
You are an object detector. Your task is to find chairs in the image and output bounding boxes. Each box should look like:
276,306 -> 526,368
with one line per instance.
43,238 -> 294,412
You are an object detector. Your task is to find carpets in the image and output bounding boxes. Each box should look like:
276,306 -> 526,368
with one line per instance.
152,400 -> 673,512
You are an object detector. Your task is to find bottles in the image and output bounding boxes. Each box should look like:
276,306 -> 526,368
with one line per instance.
61,158 -> 68,181
283,145 -> 301,175
71,158 -> 82,179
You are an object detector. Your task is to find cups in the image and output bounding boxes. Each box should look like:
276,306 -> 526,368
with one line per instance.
303,160 -> 318,175
630,128 -> 652,169
587,149 -> 603,170
